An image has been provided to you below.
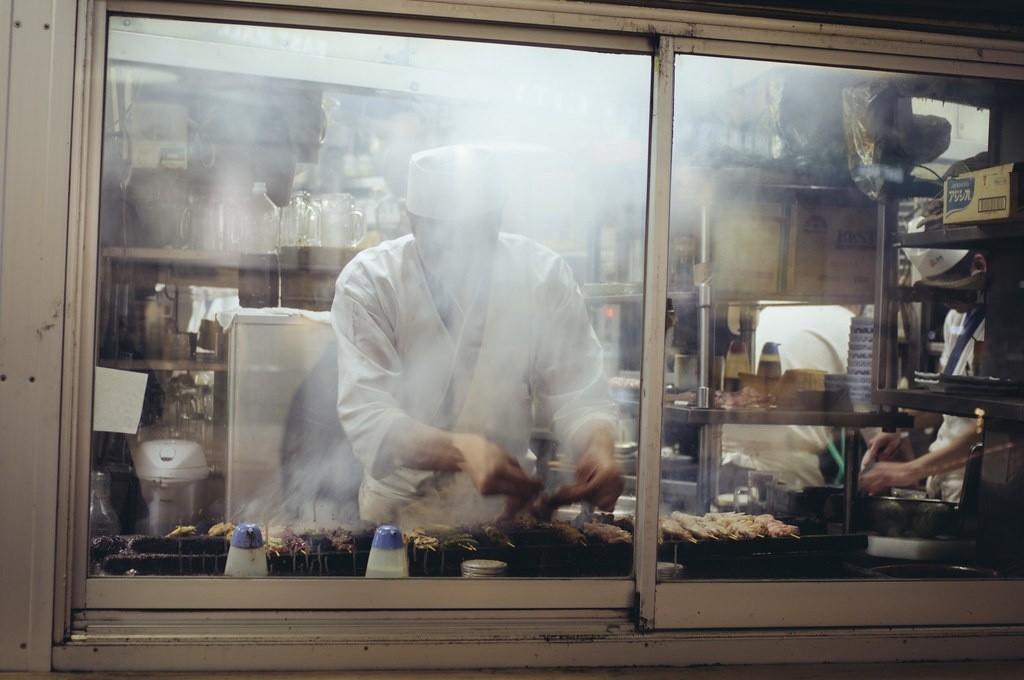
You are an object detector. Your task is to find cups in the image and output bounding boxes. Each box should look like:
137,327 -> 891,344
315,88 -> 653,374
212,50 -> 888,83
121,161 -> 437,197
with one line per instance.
321,193 -> 366,247
192,176 -> 242,253
285,190 -> 321,245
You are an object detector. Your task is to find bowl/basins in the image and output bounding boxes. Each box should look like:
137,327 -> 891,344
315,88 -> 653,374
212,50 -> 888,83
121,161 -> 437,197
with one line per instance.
825,317 -> 881,412
870,496 -> 955,537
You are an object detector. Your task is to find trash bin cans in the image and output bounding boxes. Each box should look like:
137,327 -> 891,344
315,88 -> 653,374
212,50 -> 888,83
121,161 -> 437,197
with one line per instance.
130,438 -> 208,536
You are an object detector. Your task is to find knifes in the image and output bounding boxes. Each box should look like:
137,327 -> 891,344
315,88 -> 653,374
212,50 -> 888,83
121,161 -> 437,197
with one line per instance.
862,443 -> 888,472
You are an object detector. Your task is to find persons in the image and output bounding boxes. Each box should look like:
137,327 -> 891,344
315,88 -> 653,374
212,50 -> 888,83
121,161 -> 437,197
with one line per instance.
330,143 -> 625,535
719,300 -> 859,513
858,215 -> 989,509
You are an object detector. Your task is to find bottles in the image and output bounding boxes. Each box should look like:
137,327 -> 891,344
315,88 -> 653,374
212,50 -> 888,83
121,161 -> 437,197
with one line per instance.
723,340 -> 751,392
364,526 -> 409,579
244,183 -> 278,254
90,472 -> 120,536
225,522 -> 267,576
757,342 -> 782,377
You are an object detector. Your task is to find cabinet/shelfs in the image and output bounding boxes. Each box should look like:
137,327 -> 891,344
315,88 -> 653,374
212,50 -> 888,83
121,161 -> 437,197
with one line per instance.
873,198 -> 1024,424
104,244 -> 342,375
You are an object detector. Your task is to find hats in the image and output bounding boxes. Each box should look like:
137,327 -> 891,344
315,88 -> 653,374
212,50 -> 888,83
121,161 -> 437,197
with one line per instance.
900,216 -> 970,279
404,141 -> 504,223
